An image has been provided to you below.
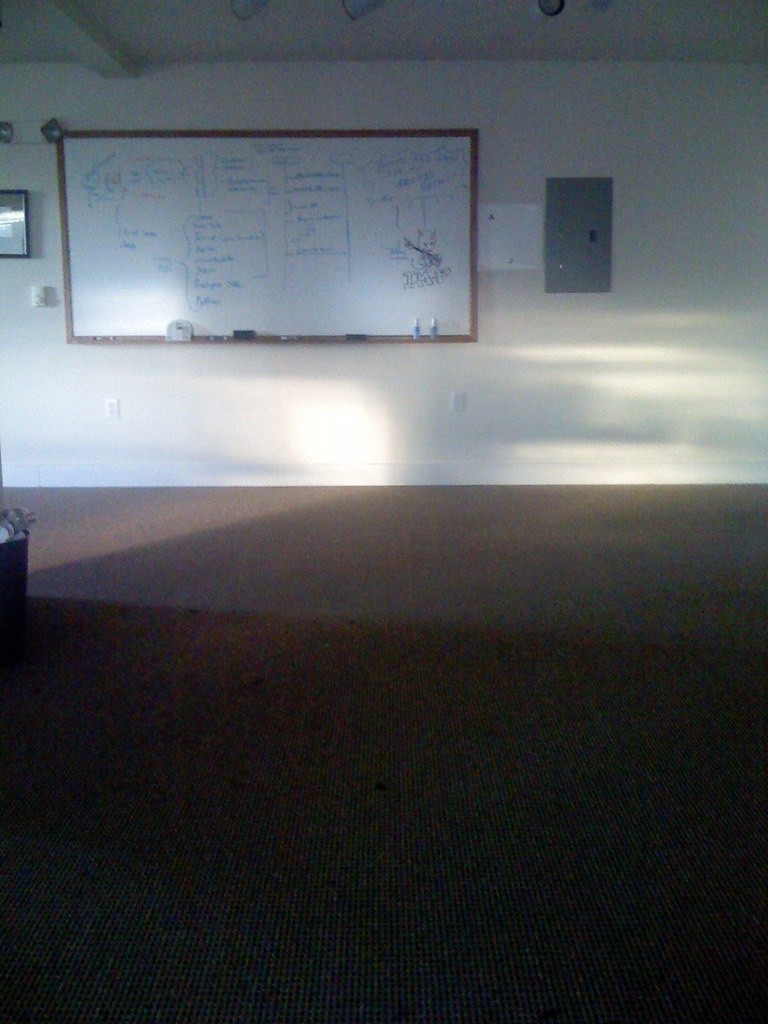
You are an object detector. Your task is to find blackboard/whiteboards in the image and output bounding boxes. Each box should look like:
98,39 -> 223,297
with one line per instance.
56,128 -> 478,345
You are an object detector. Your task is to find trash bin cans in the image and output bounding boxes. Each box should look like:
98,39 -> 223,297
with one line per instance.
0,528 -> 31,668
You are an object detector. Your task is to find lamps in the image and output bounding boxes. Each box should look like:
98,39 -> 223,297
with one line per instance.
342,0 -> 386,20
538,0 -> 567,16
39,118 -> 65,144
229,0 -> 272,21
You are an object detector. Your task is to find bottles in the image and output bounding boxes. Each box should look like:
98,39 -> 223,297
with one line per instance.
0,508 -> 36,532
0,514 -> 14,544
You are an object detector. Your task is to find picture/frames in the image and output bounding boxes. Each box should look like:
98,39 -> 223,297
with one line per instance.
0,189 -> 31,260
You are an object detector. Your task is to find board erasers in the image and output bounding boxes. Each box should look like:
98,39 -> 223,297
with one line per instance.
346,334 -> 368,342
230,330 -> 256,339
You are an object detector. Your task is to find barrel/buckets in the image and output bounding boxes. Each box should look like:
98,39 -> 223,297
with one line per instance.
0,530 -> 30,615
